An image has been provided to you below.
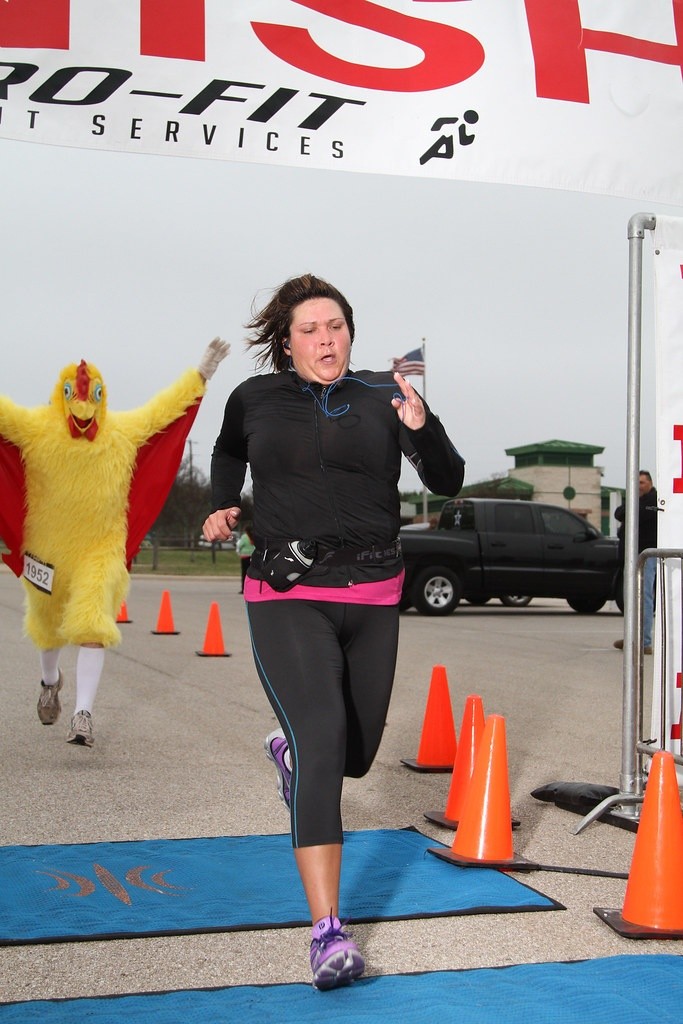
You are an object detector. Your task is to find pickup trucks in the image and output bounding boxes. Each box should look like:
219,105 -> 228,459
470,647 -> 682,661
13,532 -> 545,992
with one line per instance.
394,496 -> 655,618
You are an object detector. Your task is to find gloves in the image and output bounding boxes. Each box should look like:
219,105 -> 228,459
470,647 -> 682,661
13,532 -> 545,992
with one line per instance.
199,337 -> 231,379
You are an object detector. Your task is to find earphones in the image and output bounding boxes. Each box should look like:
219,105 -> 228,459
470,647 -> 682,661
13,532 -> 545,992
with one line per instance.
283,341 -> 291,350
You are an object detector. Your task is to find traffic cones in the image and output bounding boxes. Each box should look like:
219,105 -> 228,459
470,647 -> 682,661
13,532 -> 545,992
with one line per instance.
592,748 -> 683,941
422,694 -> 524,831
398,663 -> 458,774
424,713 -> 542,872
115,600 -> 133,624
194,600 -> 234,658
150,588 -> 181,636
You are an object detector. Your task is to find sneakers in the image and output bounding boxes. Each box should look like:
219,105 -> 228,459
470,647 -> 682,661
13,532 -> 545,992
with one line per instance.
311,906 -> 365,991
67,710 -> 95,748
264,727 -> 293,812
37,669 -> 62,725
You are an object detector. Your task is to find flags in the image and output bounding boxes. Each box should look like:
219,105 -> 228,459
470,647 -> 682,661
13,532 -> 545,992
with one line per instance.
392,347 -> 424,378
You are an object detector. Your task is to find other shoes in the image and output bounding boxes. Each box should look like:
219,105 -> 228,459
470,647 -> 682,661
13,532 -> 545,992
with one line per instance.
614,639 -> 624,649
643,646 -> 652,654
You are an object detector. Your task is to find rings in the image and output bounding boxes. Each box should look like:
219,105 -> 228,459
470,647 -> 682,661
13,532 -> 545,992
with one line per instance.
412,398 -> 417,402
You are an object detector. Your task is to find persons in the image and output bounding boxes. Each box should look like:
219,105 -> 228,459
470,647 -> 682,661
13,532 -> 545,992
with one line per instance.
613,472 -> 657,655
0,337 -> 232,748
235,531 -> 256,594
203,274 -> 466,992
425,515 -> 438,531
